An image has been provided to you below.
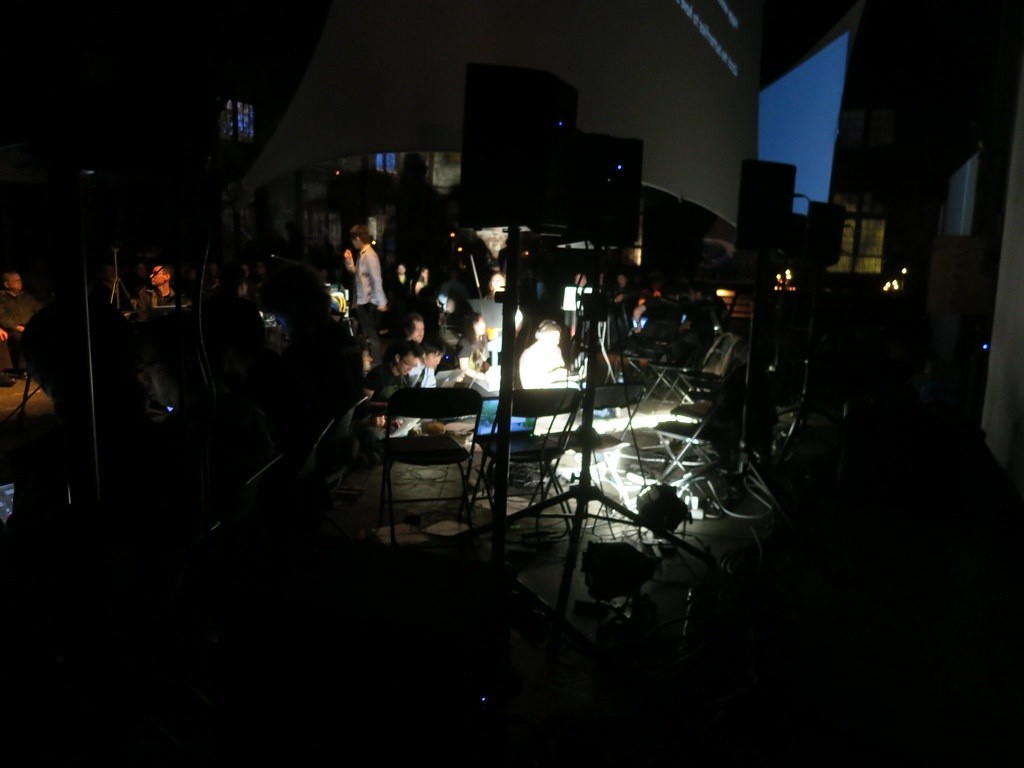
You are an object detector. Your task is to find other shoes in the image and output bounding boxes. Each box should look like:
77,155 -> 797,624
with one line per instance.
0,372 -> 17,387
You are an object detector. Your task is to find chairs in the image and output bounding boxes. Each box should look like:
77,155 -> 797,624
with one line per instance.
199,301 -> 775,555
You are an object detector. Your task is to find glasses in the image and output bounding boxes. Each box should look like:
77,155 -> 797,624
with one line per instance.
401,357 -> 417,369
8,279 -> 24,285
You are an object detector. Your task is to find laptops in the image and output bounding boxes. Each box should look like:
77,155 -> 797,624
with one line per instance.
474,396 -> 561,456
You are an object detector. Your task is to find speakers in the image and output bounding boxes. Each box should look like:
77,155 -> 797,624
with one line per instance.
735,160 -> 796,251
803,202 -> 847,264
558,132 -> 644,244
460,64 -> 578,236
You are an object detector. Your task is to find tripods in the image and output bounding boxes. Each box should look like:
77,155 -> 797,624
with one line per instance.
110,247 -> 131,310
445,226 -> 850,672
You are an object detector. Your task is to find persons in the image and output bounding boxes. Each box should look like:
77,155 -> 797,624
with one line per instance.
0,246 -> 350,421
342,225 -> 730,456
